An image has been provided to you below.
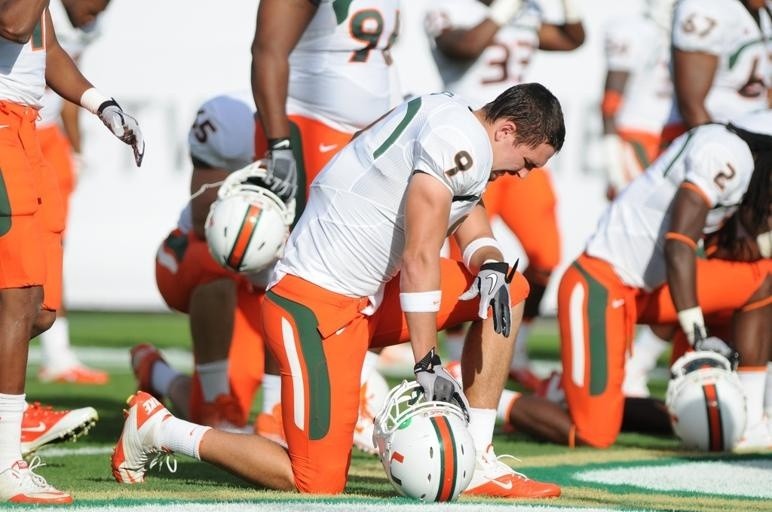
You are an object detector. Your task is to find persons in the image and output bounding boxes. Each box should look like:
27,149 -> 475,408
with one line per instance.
1,1 -> 149,509
36,1 -> 117,392
110,76 -> 566,503
137,0 -> 769,456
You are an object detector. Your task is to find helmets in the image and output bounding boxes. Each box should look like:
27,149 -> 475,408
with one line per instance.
664,351 -> 748,453
372,379 -> 477,503
204,157 -> 297,276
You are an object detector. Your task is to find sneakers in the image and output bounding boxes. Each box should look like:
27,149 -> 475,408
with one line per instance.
458,443 -> 561,502
191,372 -> 244,436
0,459 -> 73,506
108,389 -> 173,484
251,402 -> 290,451
37,353 -> 110,386
352,399 -> 380,457
131,342 -> 170,407
19,401 -> 99,461
509,363 -> 569,411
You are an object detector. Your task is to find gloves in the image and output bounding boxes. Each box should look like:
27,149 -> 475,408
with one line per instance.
260,137 -> 298,203
406,345 -> 474,423
96,95 -> 146,168
456,256 -> 520,339
684,321 -> 741,372
